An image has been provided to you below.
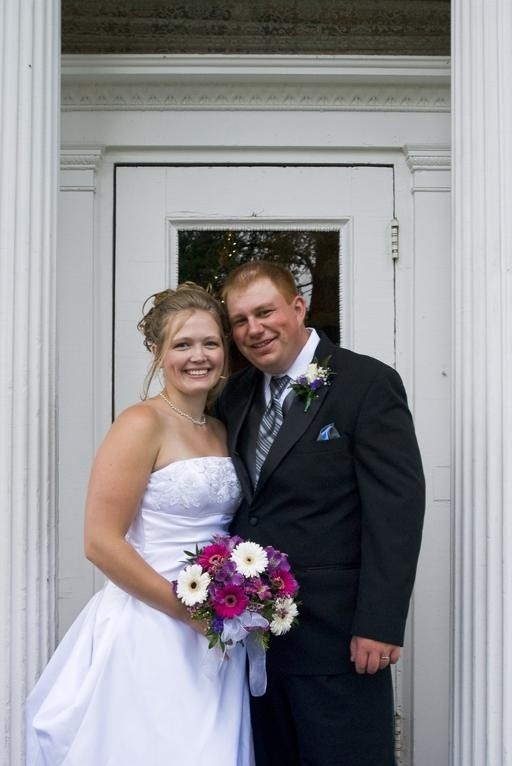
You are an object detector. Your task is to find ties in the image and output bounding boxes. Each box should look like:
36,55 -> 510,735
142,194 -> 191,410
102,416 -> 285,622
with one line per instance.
254,372 -> 291,488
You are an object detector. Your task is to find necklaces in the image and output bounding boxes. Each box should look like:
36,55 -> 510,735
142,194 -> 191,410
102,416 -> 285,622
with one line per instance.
159,390 -> 209,425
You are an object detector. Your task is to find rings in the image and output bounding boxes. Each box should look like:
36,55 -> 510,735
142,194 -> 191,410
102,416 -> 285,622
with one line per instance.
379,656 -> 391,662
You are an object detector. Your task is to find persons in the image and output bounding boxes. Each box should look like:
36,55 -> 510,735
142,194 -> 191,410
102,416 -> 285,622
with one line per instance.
204,260 -> 426,766
19,280 -> 258,765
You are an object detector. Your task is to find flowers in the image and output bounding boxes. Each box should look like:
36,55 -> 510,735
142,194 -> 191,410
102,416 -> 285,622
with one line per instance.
288,355 -> 337,413
172,531 -> 303,653
